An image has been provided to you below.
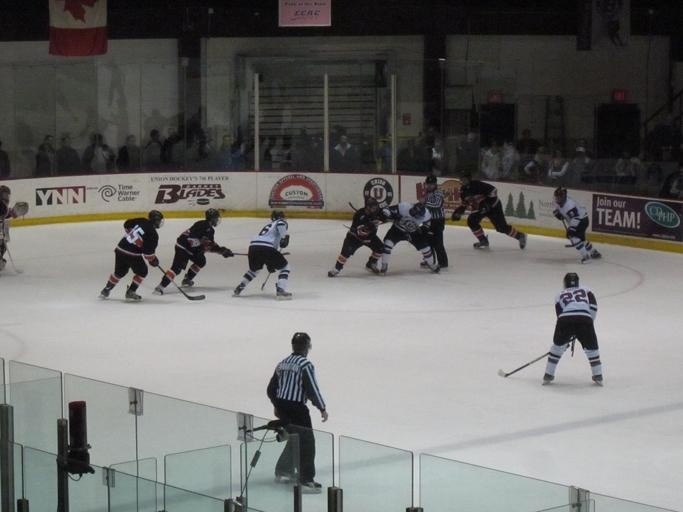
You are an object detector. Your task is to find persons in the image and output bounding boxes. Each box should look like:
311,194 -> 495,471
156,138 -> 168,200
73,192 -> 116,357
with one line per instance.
379,202 -> 440,272
0,186 -> 11,273
236,209 -> 292,296
422,174 -> 447,269
544,273 -> 603,381
35,135 -> 683,202
553,188 -> 601,261
1,141 -> 10,179
329,197 -> 385,276
155,208 -> 233,294
267,334 -> 328,488
102,209 -> 164,299
451,175 -> 526,250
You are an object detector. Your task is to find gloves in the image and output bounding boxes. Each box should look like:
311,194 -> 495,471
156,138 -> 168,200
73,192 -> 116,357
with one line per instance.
220,248 -> 234,257
279,236 -> 289,248
150,257 -> 159,267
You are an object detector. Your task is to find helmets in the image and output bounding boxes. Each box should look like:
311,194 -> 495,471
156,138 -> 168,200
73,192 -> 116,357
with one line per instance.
565,273 -> 579,288
271,210 -> 285,220
554,187 -> 567,204
205,208 -> 220,227
366,197 -> 380,216
291,331 -> 311,354
411,202 -> 426,218
148,210 -> 163,228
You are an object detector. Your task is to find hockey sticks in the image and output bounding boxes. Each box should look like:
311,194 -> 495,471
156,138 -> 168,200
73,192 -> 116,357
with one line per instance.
233,252 -> 290,256
498,335 -> 577,378
562,219 -> 575,248
2,240 -> 23,274
156,264 -> 206,300
260,248 -> 283,292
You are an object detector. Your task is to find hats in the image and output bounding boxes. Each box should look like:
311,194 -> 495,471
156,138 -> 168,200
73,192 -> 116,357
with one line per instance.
426,175 -> 436,185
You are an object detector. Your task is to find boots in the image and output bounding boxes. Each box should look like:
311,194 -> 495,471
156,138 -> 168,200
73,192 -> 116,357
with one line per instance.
474,235 -> 489,246
328,269 -> 339,277
126,290 -> 141,299
182,279 -> 194,285
277,287 -> 292,295
592,375 -> 603,381
366,257 -> 380,273
156,285 -> 164,295
101,289 -> 110,297
544,374 -> 555,381
236,285 -> 245,292
516,233 -> 525,249
381,263 -> 388,273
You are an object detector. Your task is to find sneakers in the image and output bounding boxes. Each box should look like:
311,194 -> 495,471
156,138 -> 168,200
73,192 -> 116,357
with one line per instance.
591,251 -> 601,259
298,477 -> 321,489
